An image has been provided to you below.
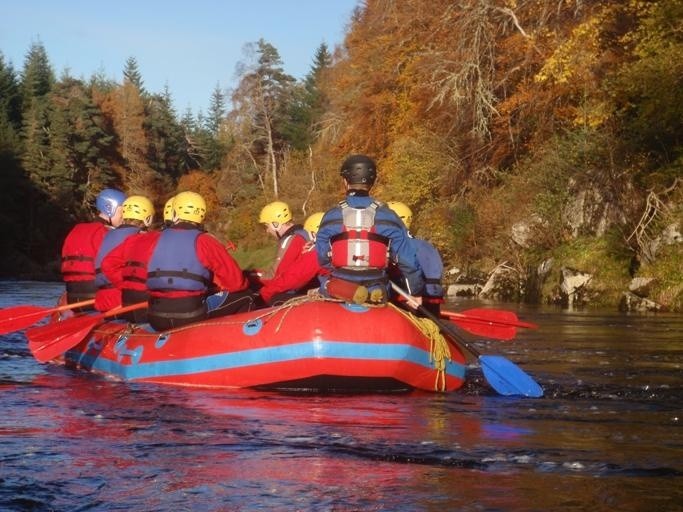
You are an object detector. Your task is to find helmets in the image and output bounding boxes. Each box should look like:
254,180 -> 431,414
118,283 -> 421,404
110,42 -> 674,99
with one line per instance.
386,201 -> 412,230
304,212 -> 325,242
259,202 -> 291,231
121,195 -> 155,227
96,189 -> 125,216
163,191 -> 206,225
340,155 -> 376,186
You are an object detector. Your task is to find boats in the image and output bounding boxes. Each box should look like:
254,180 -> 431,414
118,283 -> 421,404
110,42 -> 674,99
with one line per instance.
49,291 -> 468,396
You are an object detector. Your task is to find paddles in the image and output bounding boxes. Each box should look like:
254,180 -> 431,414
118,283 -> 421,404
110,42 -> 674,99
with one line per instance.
0,298 -> 150,365
388,279 -> 544,398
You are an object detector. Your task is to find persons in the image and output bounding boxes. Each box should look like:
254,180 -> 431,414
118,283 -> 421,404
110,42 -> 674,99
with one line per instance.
246,200 -> 444,318
60,188 -> 259,332
315,155 -> 424,310
